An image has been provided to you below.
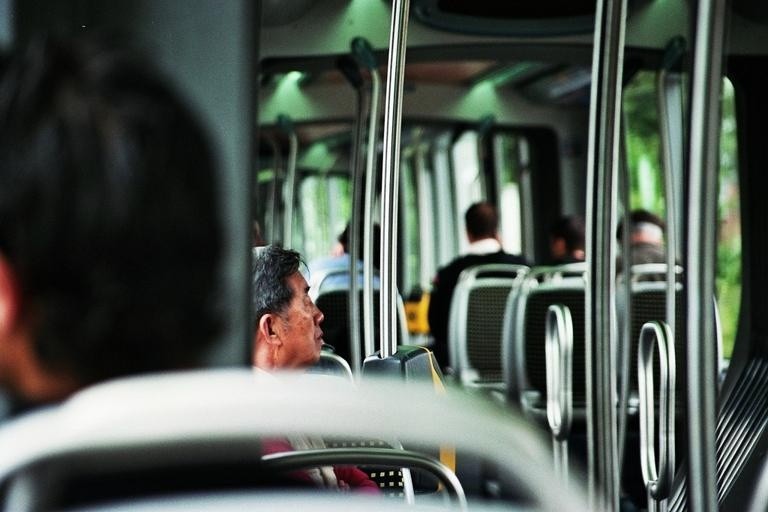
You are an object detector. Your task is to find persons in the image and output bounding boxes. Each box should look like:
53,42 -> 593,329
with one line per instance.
253,239 -> 379,496
0,30 -> 323,512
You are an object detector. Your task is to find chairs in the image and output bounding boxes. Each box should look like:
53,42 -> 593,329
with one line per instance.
310,267 -> 437,383
446,261 -> 723,441
1,380 -> 591,512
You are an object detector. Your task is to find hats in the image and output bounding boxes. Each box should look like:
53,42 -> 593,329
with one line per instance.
615,208 -> 667,240
